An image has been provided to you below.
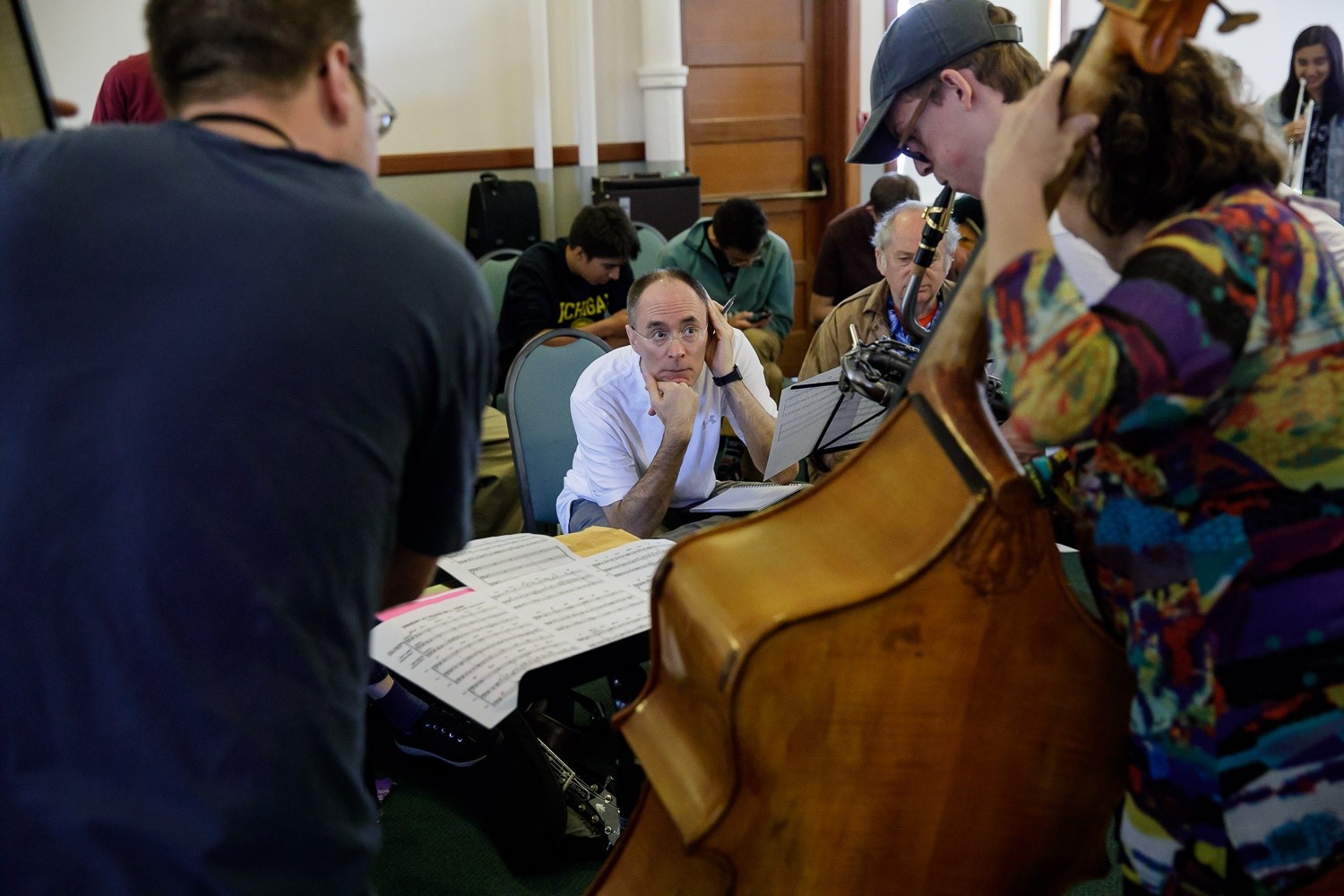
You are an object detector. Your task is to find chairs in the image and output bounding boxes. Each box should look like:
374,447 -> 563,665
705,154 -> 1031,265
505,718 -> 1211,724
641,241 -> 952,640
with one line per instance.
505,328 -> 614,535
476,249 -> 524,326
629,221 -> 669,281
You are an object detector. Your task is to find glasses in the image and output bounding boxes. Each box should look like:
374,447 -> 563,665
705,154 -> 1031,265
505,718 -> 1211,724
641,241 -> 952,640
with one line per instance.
630,324 -> 710,346
318,59 -> 397,137
898,62 -> 972,163
725,244 -> 766,267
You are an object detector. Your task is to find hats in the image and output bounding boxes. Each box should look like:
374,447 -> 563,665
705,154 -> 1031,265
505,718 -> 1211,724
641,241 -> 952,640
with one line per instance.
845,1 -> 1023,163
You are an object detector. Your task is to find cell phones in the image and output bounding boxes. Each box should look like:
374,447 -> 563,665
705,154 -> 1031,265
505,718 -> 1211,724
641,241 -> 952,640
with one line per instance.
746,312 -> 770,324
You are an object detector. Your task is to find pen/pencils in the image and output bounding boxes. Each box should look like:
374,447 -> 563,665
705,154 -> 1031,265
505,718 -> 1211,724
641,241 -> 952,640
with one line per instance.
721,295 -> 737,315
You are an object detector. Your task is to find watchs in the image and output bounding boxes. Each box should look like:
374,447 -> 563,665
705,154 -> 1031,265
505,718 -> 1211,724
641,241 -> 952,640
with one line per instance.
712,363 -> 743,386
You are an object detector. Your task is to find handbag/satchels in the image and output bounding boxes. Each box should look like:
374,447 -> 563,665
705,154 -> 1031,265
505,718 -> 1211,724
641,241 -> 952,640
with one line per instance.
464,173 -> 543,260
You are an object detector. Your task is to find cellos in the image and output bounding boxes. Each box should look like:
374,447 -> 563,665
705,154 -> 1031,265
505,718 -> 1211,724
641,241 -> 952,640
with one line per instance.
582,0 -> 1261,896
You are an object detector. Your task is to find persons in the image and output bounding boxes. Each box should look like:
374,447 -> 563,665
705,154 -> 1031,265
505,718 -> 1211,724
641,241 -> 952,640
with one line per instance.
556,268 -> 799,538
1260,25 -> 1343,222
92,50 -> 166,124
845,0 -> 1119,309
0,0 -> 492,896
798,173 -> 985,483
981,26 -> 1343,896
493,203 -> 642,392
658,198 -> 795,482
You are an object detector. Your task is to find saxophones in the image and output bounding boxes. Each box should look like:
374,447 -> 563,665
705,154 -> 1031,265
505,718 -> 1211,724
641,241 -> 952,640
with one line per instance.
838,182 -> 1013,426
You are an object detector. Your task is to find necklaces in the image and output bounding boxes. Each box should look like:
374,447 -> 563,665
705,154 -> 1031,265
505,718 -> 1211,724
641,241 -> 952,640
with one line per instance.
192,112 -> 294,144
